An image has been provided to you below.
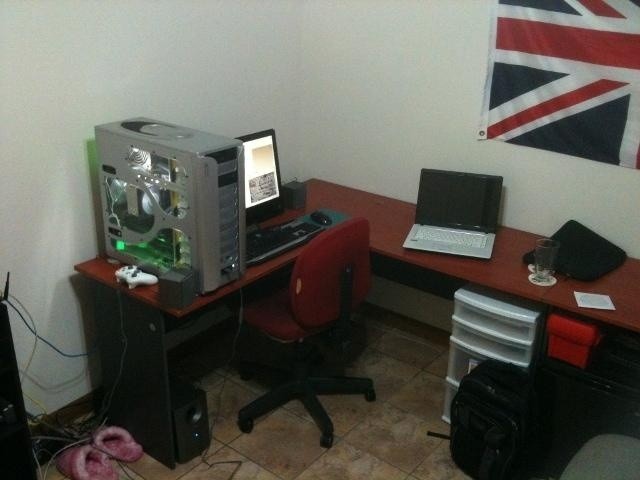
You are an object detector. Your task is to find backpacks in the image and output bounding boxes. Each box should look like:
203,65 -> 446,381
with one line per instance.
449,359 -> 553,480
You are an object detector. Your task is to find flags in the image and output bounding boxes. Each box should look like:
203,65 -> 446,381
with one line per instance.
476,0 -> 638,172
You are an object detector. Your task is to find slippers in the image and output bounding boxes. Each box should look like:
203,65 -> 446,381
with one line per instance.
56,445 -> 119,480
90,425 -> 144,462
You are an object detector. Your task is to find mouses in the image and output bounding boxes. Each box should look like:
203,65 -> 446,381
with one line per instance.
311,212 -> 332,225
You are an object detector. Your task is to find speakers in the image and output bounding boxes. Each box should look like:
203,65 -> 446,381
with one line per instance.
283,182 -> 305,210
168,383 -> 210,463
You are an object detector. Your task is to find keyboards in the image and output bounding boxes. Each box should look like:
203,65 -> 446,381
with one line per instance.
246,219 -> 326,268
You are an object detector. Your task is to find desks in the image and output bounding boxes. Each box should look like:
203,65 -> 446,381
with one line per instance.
73,179 -> 640,468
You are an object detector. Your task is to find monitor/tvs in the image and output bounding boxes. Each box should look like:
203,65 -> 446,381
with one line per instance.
235,128 -> 282,234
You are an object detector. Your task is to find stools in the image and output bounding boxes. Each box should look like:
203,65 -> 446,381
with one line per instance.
563,434 -> 640,480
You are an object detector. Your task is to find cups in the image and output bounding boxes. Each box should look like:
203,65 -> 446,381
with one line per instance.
534,238 -> 560,283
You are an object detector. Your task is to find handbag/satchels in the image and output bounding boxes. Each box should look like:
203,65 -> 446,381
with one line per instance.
522,219 -> 627,282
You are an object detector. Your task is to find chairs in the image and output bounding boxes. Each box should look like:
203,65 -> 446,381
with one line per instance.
236,217 -> 377,448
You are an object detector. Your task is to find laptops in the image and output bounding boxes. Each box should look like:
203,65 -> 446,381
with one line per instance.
403,168 -> 503,260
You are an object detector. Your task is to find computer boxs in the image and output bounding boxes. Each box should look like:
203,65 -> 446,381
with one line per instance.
95,117 -> 246,294
